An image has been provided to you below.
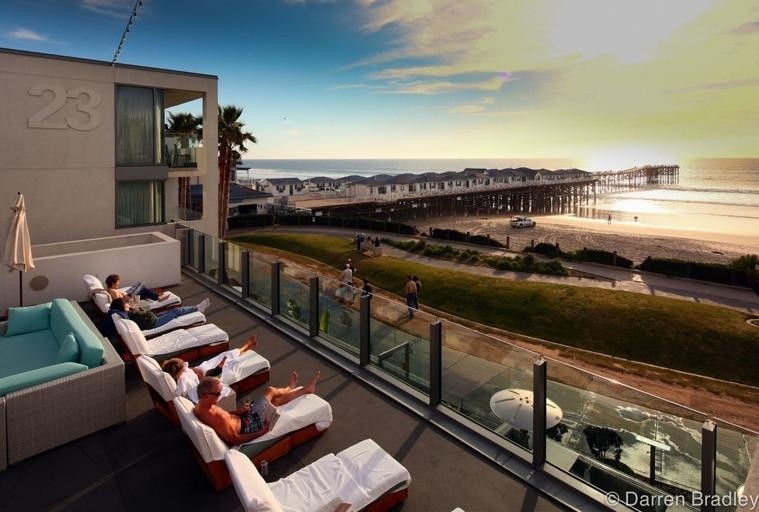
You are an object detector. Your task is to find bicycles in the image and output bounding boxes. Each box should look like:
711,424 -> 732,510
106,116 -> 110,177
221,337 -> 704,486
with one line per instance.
340,279 -> 359,295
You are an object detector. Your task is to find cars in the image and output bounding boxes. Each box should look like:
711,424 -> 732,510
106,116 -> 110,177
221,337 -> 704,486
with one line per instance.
510,215 -> 536,229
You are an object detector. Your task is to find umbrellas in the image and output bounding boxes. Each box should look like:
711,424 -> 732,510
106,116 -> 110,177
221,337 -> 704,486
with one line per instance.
3,191 -> 36,305
490,388 -> 563,432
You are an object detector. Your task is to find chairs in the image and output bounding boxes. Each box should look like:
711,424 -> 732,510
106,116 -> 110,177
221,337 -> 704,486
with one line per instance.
172,385 -> 332,492
173,143 -> 191,167
137,347 -> 272,426
82,274 -> 230,367
226,438 -> 411,512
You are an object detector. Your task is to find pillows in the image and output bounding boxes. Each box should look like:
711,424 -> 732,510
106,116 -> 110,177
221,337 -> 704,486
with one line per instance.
57,332 -> 79,365
5,301 -> 52,337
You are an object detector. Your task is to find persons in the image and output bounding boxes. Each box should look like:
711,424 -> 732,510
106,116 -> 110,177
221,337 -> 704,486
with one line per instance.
162,334 -> 258,385
193,369 -> 321,447
354,232 -> 380,252
106,273 -> 171,303
608,214 -> 612,224
406,274 -> 423,319
108,296 -> 211,330
335,258 -> 373,307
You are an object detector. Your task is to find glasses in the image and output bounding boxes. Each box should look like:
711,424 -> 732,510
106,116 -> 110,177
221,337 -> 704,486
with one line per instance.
203,390 -> 221,396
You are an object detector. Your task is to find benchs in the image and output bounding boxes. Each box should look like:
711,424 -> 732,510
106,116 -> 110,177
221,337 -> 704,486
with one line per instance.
360,243 -> 382,257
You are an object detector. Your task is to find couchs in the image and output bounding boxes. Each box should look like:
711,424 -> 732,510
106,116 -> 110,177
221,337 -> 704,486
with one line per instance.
1,296 -> 127,472
